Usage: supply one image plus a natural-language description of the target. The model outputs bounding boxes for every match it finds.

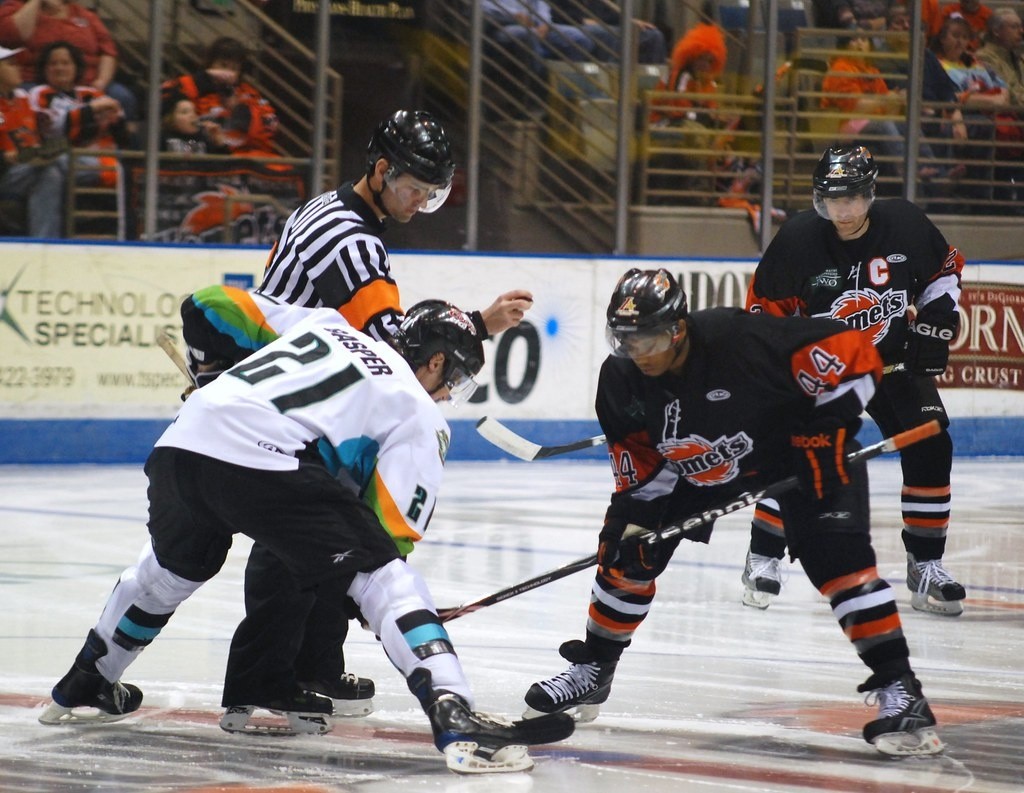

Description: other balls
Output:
[514,297,534,312]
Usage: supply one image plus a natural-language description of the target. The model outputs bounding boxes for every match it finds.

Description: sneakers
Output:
[521,640,624,722]
[407,668,534,774]
[219,687,333,736]
[302,671,376,718]
[856,667,946,757]
[905,551,966,616]
[38,627,144,724]
[740,547,786,609]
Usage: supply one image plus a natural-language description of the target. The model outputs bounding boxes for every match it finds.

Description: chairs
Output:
[405,1,855,202]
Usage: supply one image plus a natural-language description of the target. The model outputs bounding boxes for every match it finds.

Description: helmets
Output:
[397,298,485,386]
[811,145,879,203]
[365,109,456,190]
[605,266,688,339]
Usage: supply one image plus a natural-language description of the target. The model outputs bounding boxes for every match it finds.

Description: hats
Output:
[1,45,25,60]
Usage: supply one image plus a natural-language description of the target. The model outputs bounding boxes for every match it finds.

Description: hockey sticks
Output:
[376,420,942,641]
[156,334,575,746]
[475,362,905,463]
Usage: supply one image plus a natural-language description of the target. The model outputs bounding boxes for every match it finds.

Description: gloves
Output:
[180,351,237,401]
[785,414,852,503]
[595,492,684,581]
[903,309,959,376]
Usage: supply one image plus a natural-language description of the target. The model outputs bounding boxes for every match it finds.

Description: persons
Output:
[221,107,533,737]
[522,268,947,758]
[741,139,965,616]
[463,0,1024,208]
[36,286,546,775]
[0,0,304,237]
